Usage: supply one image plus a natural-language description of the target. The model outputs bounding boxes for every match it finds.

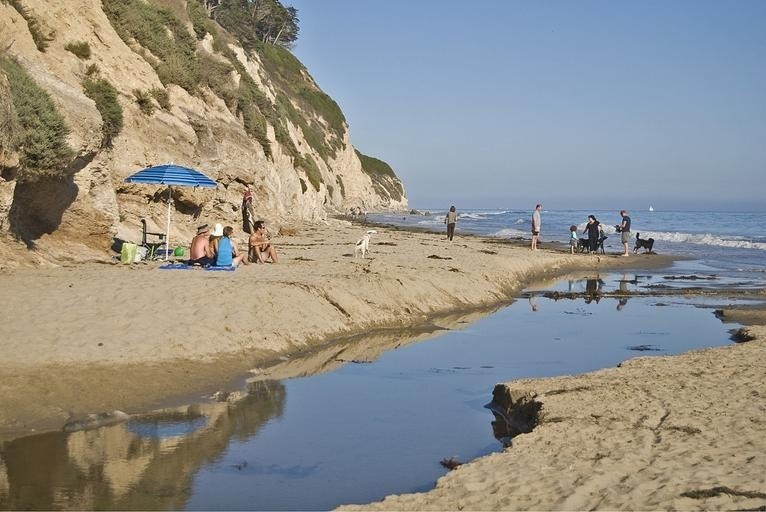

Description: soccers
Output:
[174,248,183,256]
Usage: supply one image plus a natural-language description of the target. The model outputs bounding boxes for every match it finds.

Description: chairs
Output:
[141,219,167,261]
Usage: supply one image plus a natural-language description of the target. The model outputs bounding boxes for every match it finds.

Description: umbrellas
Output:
[123,161,218,260]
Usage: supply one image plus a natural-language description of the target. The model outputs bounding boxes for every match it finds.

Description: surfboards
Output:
[245,190,255,232]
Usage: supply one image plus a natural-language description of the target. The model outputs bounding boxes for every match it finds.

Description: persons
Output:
[568,224,580,255]
[247,220,280,265]
[209,222,223,266]
[530,203,543,251]
[188,222,215,266]
[443,205,457,241]
[584,280,597,304]
[215,226,249,269]
[614,272,630,311]
[528,296,538,312]
[582,214,603,255]
[618,209,631,257]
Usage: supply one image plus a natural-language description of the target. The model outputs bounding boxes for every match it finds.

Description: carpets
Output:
[157,261,239,272]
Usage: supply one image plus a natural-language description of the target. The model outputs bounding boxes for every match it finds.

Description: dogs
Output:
[633,232,654,256]
[354,234,370,258]
[579,238,590,253]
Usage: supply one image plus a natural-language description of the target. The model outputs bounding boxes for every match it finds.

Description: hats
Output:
[196,223,223,237]
